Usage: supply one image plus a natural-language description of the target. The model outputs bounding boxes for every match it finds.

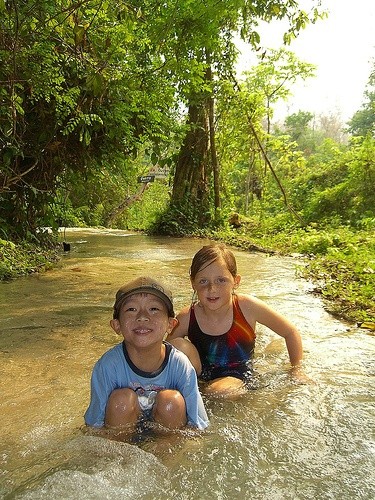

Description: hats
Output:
[113,276,176,317]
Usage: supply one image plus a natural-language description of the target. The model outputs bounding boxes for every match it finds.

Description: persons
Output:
[83,277,210,433]
[165,241,303,399]
